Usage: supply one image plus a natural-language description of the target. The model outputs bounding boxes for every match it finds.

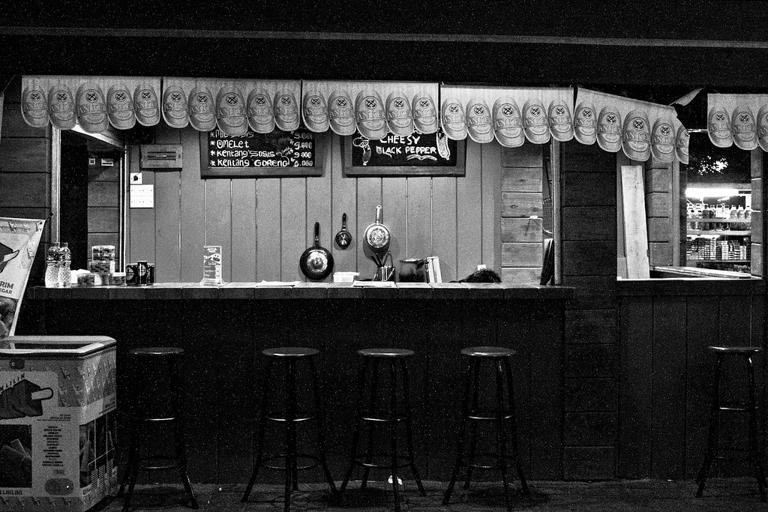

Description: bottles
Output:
[45,242,71,289]
[687,203,752,260]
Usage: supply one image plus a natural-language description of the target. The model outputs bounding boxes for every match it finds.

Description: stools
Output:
[240,347,337,512]
[440,344,530,512]
[337,348,427,512]
[120,346,199,512]
[695,344,768,503]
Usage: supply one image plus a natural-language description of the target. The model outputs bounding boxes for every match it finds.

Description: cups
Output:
[78,273,95,286]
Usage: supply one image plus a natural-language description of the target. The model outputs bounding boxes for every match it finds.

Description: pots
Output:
[300,205,390,281]
[399,258,426,281]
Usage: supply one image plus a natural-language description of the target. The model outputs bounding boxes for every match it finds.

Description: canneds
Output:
[126,260,155,287]
